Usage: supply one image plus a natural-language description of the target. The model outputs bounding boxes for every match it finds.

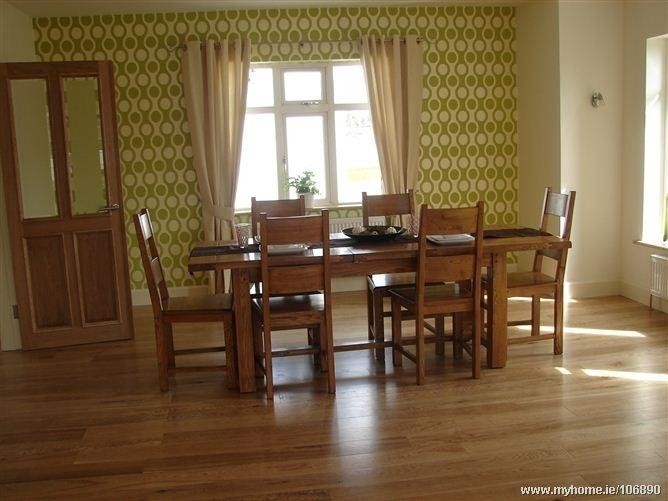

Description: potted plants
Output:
[286,170,320,210]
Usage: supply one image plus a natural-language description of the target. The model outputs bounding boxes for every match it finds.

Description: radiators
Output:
[226,216,386,296]
[648,255,668,311]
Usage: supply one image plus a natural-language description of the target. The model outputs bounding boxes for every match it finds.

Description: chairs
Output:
[386,201,485,387]
[251,196,319,348]
[361,190,447,362]
[132,208,239,393]
[456,184,577,369]
[248,210,338,399]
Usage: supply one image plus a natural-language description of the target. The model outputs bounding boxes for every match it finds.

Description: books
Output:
[425,234,474,244]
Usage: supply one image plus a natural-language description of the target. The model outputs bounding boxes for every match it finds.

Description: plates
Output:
[341,225,406,240]
[425,234,476,246]
[258,244,308,254]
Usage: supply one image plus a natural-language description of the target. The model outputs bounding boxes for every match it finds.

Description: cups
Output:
[233,222,250,249]
